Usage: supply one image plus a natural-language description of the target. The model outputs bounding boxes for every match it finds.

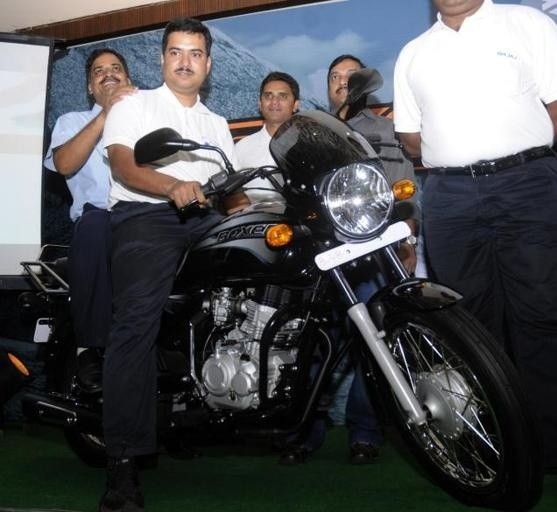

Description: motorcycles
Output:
[0,66,531,504]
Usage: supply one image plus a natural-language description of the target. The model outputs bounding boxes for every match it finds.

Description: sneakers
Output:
[279,441,319,466]
[98,456,145,512]
[347,441,385,465]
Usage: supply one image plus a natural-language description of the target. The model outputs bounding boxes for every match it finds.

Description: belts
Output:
[426,146,554,176]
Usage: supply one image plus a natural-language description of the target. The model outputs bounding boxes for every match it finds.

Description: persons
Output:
[385,1,556,484]
[95,11,261,512]
[233,67,305,202]
[278,51,423,474]
[41,46,146,468]
